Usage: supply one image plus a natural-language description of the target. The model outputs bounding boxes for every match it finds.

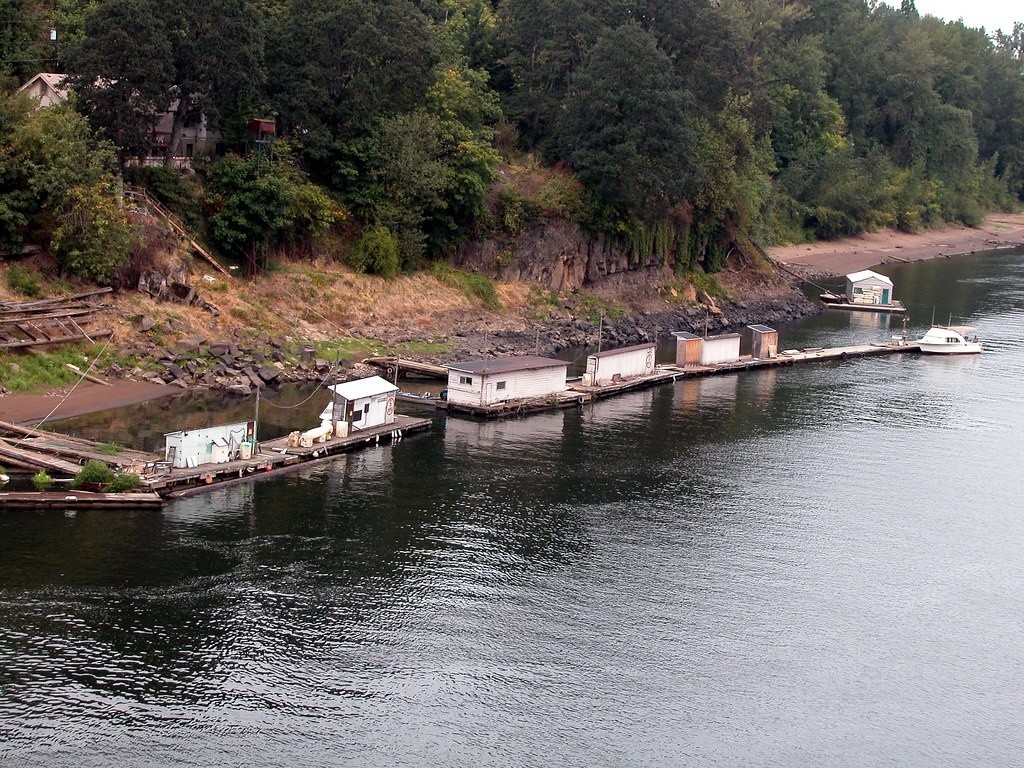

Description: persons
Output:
[964,335,977,343]
[125,188,142,203]
[172,227,185,242]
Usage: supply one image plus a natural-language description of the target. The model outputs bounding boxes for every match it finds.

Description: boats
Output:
[916,325,984,356]
